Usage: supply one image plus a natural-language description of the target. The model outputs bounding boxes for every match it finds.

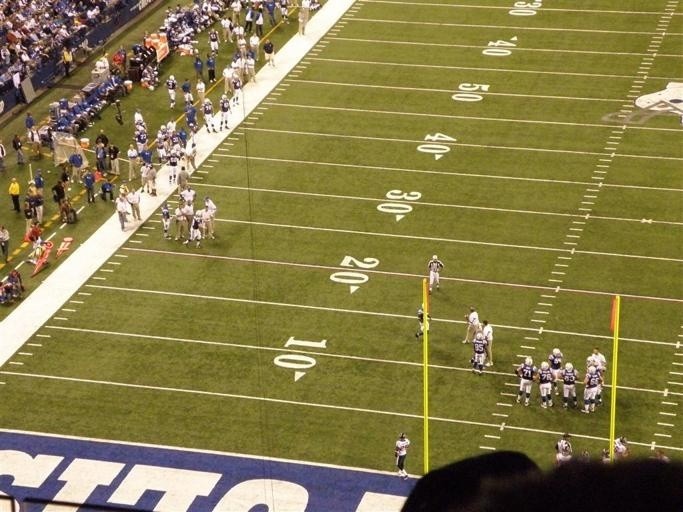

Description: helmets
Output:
[476,333,597,374]
[399,432,405,439]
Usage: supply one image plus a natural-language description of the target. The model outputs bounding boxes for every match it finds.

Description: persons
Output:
[395,433,410,481]
[555,433,630,481]
[509,347,607,415]
[416,303,431,339]
[0,1,321,303]
[428,255,444,295]
[656,450,670,463]
[462,307,494,376]
